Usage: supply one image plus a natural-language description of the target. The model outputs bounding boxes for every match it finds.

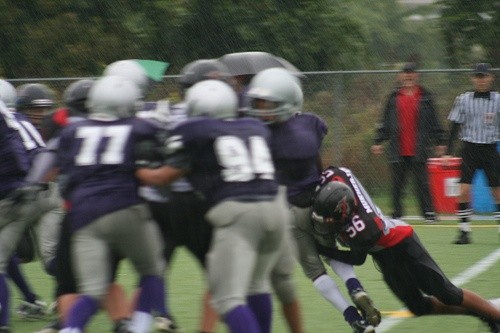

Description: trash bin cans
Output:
[427,157,463,214]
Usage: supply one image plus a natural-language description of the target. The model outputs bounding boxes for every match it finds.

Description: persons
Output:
[371,62,445,223]
[293,167,500,333]
[0,59,380,333]
[443,61,500,244]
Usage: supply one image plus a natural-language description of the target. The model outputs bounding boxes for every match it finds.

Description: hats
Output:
[472,62,493,75]
[401,64,416,73]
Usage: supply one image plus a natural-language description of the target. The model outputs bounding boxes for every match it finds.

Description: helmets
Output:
[85,75,142,122]
[176,59,234,85]
[311,180,355,235]
[103,60,150,98]
[183,79,239,121]
[0,79,15,104]
[14,84,57,113]
[243,67,304,125]
[63,79,92,110]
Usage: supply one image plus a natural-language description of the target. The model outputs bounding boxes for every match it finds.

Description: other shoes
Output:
[452,231,469,244]
[424,211,436,220]
[351,288,381,327]
[351,319,376,333]
[155,316,174,332]
[47,300,58,315]
[17,301,45,320]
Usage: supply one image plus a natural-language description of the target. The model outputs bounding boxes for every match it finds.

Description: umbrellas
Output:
[219,52,307,77]
